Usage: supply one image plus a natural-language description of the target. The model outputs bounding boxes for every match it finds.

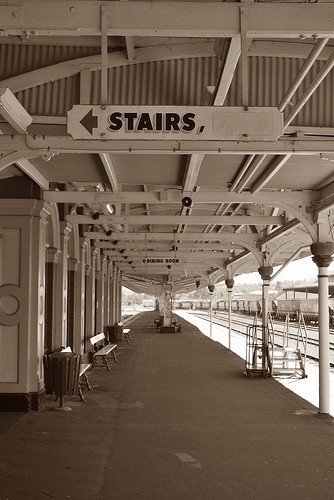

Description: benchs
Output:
[153,316,182,333]
[60,345,93,402]
[89,332,119,371]
[118,321,133,344]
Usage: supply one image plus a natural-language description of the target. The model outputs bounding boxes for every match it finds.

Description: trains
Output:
[142,299,334,324]
